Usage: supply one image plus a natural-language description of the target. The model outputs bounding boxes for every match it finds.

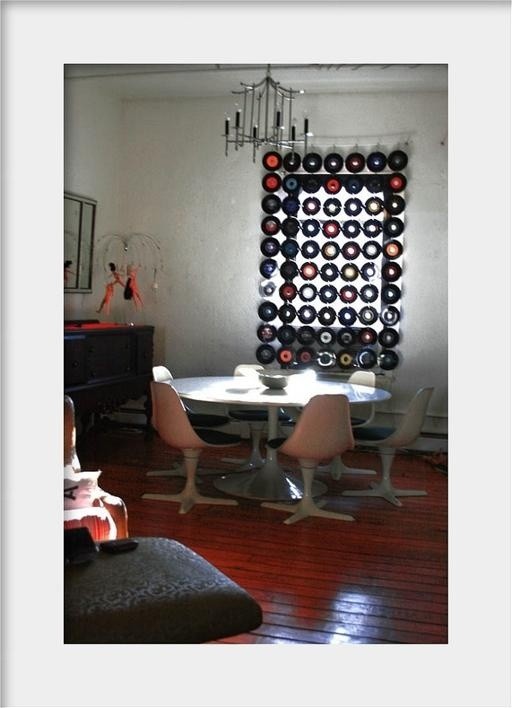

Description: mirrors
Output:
[65,193,99,294]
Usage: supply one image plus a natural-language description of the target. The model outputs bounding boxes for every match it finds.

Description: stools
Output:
[65,536,264,642]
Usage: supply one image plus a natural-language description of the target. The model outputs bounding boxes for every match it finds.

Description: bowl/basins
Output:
[254,368,306,390]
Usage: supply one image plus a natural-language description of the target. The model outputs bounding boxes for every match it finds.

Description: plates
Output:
[256,148,407,372]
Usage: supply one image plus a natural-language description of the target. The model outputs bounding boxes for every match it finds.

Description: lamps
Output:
[220,64,314,165]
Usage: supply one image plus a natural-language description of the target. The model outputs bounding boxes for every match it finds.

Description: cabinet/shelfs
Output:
[64,318,155,445]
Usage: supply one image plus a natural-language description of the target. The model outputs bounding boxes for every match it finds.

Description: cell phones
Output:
[100,539,138,553]
